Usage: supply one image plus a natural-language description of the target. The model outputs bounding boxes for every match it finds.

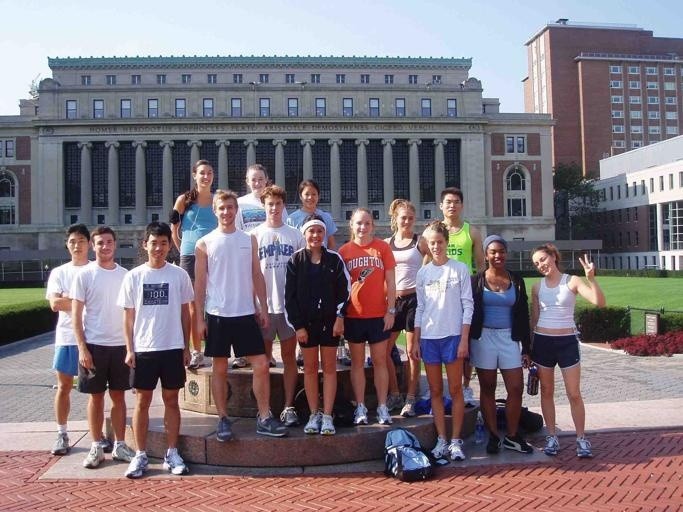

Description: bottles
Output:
[527,367,540,395]
[337,336,348,359]
[473,411,485,443]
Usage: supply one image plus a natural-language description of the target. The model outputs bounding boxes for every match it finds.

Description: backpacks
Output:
[384,427,436,482]
[414,397,452,415]
[494,399,543,432]
[292,384,359,426]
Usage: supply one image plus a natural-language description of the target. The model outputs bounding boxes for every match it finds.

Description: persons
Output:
[170,159,219,368]
[469,235,535,454]
[288,179,352,365]
[423,187,487,408]
[381,198,433,417]
[232,163,289,368]
[117,222,196,479]
[411,220,474,462]
[45,224,111,454]
[283,214,351,436]
[249,186,305,427]
[529,244,606,458]
[337,207,397,426]
[194,190,289,443]
[69,225,136,468]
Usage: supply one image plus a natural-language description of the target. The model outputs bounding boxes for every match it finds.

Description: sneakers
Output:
[386,394,404,411]
[448,439,466,461]
[232,357,246,368]
[544,436,560,455]
[304,412,324,433]
[337,349,351,365]
[112,444,136,462]
[320,415,336,435]
[270,357,276,367]
[83,446,105,468]
[280,406,300,426]
[376,406,393,425]
[430,437,451,458]
[503,435,533,453]
[576,437,593,458]
[188,351,204,369]
[126,453,149,478]
[163,451,190,474]
[487,434,502,453]
[51,433,69,454]
[463,387,475,407]
[256,415,289,437]
[296,350,303,366]
[353,404,368,424]
[400,398,416,416]
[217,416,232,442]
[100,436,110,451]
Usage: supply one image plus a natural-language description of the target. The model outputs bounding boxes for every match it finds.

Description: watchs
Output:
[336,312,344,317]
[386,307,396,313]
